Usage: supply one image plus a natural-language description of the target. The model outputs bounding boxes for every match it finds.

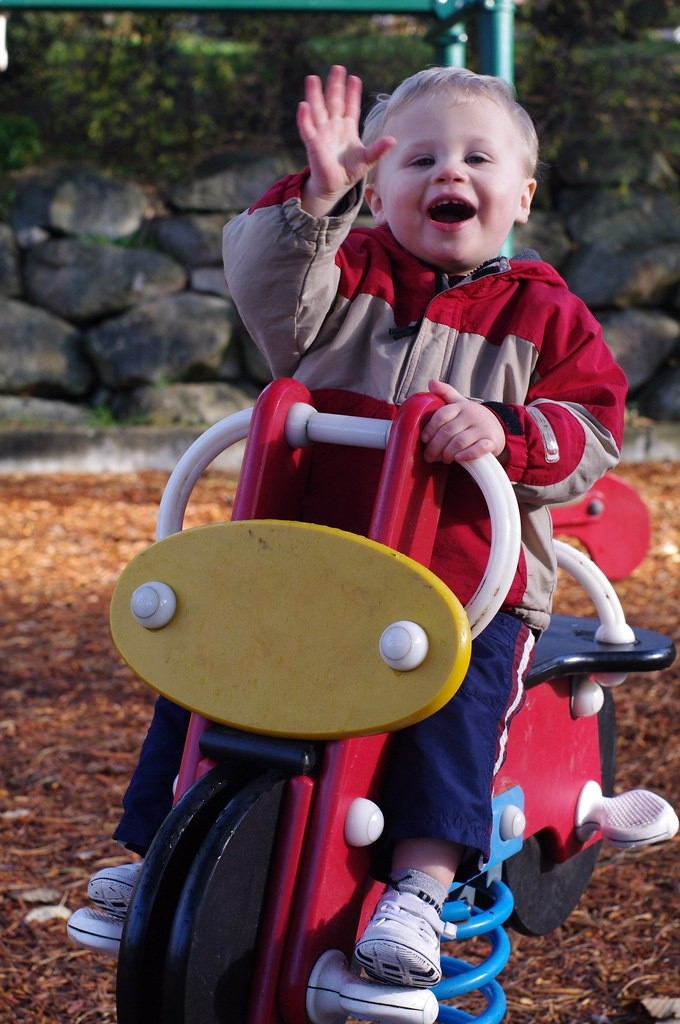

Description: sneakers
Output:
[85,862,144,919]
[352,891,458,990]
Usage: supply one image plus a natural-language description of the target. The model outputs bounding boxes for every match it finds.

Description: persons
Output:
[88,66,628,986]
